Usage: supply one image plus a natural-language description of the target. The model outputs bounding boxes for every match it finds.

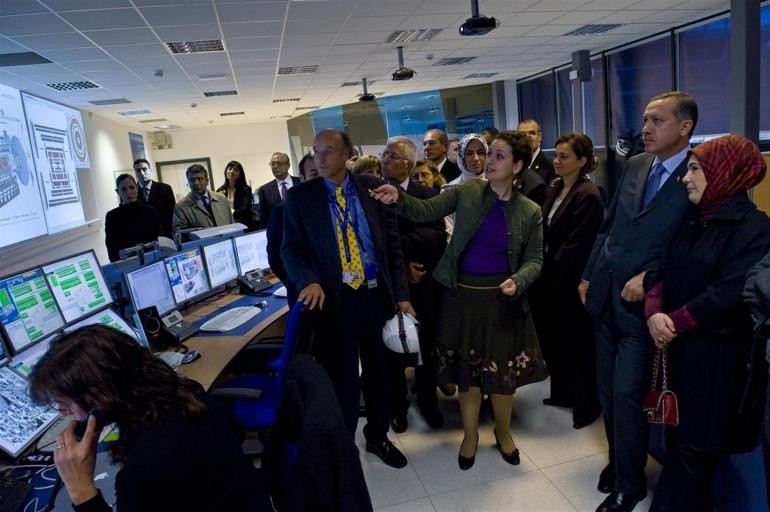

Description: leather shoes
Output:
[494,427,520,465]
[391,414,407,433]
[597,464,616,493]
[366,436,407,468]
[420,405,444,429]
[458,433,479,470]
[595,484,647,511]
[439,382,455,396]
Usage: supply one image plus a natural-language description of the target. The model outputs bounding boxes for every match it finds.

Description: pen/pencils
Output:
[367,185,402,206]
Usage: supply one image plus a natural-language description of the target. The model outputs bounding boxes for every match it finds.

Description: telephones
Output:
[159,308,199,345]
[237,267,273,292]
[73,405,108,443]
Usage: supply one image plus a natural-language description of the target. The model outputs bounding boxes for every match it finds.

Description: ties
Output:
[282,181,287,199]
[143,187,149,201]
[334,186,366,291]
[642,162,666,210]
[201,194,212,214]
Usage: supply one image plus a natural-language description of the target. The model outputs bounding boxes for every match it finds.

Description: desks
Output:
[35,273,291,511]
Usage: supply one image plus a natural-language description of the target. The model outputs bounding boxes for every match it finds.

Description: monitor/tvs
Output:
[0,248,146,458]
[200,236,241,290]
[100,226,205,308]
[232,228,273,277]
[164,244,213,310]
[120,258,180,318]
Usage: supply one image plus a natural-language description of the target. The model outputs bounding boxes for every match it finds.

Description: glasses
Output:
[269,161,287,167]
[378,152,410,163]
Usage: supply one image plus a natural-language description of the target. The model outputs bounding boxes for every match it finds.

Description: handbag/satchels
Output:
[641,389,680,426]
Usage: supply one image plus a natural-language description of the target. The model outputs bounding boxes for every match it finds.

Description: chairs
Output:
[212,289,313,426]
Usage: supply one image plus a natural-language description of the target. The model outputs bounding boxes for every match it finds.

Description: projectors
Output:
[392,68,414,80]
[459,18,495,36]
[359,93,374,101]
